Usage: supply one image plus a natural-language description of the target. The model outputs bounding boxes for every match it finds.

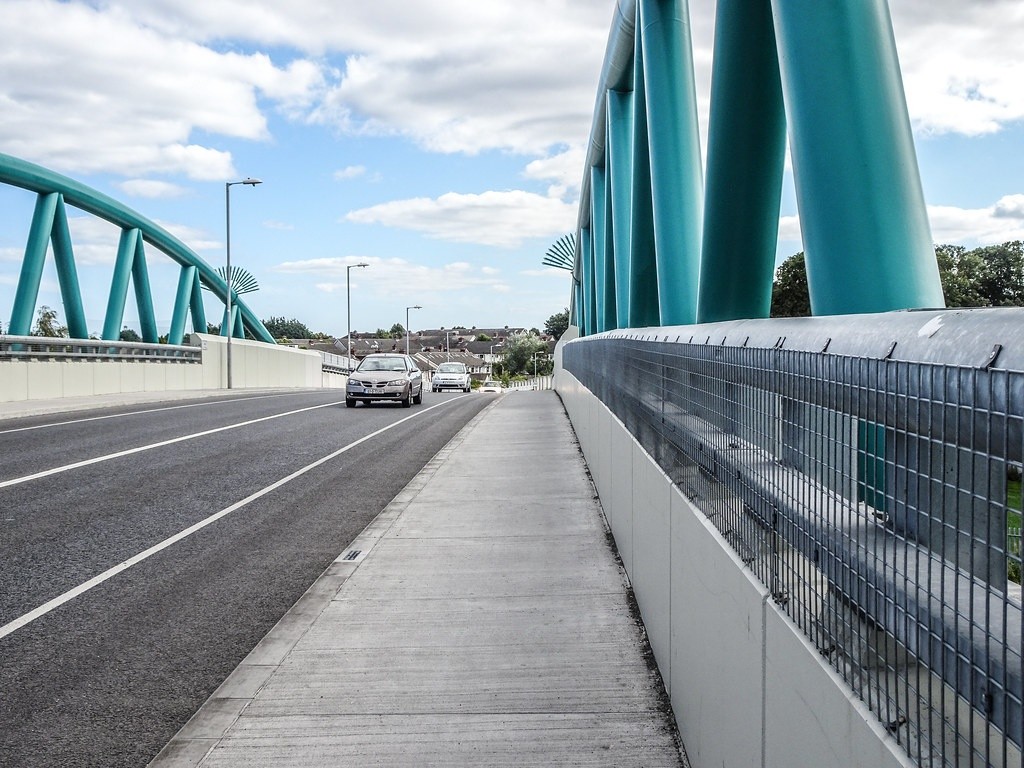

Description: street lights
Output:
[406,305,423,355]
[347,261,370,376]
[535,351,545,379]
[225,177,264,390]
[446,330,459,362]
[490,343,502,382]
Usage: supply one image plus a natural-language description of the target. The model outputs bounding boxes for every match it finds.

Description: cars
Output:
[346,352,423,408]
[431,361,471,393]
